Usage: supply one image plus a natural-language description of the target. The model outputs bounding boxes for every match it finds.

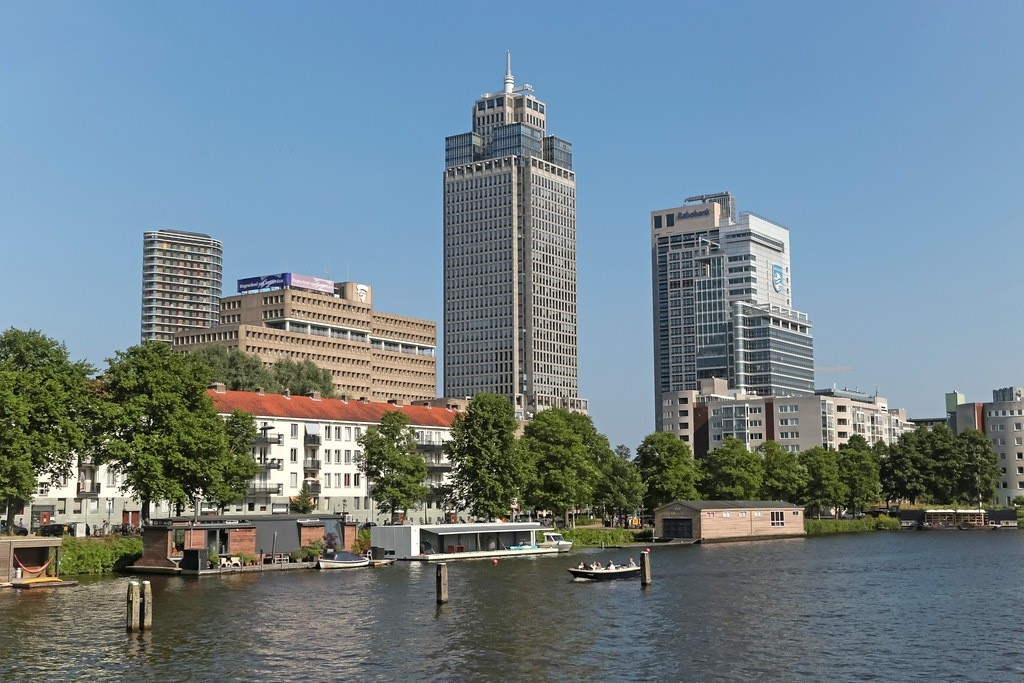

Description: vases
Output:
[257,562,260,565]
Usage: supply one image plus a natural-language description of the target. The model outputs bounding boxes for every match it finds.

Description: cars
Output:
[35,524,74,537]
[111,525,131,536]
[0,519,28,536]
[556,520,572,529]
[839,506,899,521]
[363,521,377,530]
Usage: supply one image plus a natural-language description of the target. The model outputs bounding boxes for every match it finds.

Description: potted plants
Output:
[302,539,324,562]
[292,550,307,562]
[323,532,338,553]
[243,555,257,566]
[352,538,369,557]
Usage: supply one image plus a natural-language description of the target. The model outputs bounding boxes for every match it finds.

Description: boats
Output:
[567,565,642,581]
[899,519,1003,531]
[318,554,371,569]
[534,531,574,552]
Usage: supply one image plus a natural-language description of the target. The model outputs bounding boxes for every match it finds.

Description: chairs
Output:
[219,558,230,567]
[230,557,240,567]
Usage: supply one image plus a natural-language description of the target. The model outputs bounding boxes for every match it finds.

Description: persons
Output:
[119,523,140,535]
[602,513,655,528]
[18,518,24,528]
[577,555,636,570]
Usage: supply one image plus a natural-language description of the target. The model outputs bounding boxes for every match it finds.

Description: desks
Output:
[447,545,465,553]
